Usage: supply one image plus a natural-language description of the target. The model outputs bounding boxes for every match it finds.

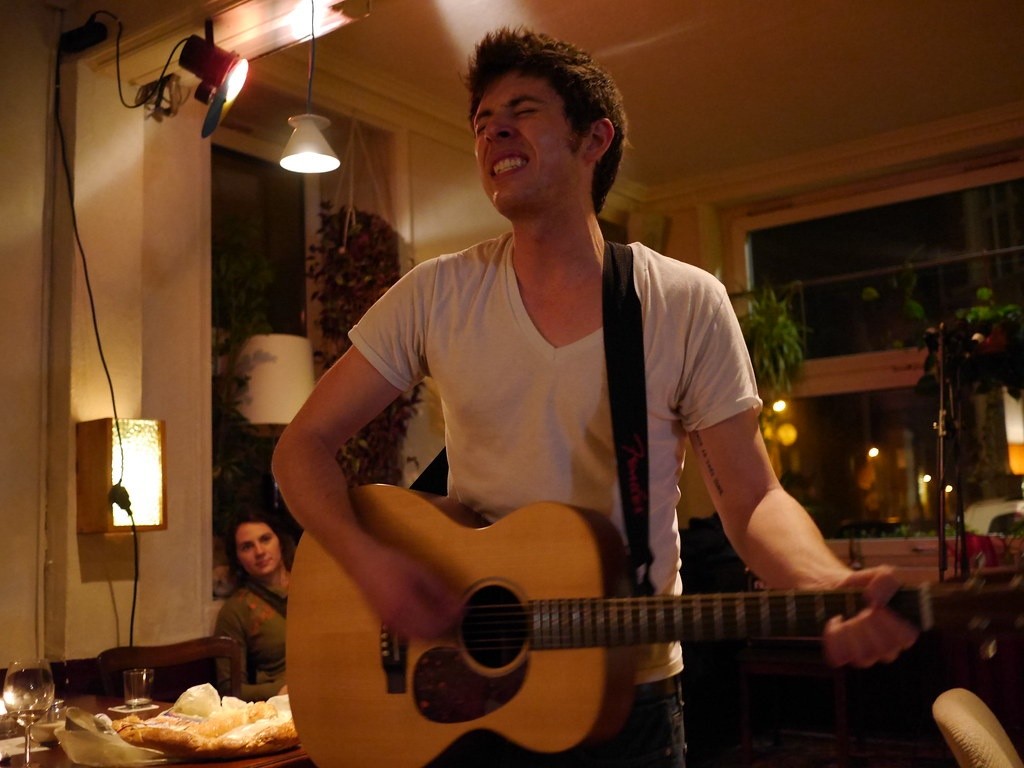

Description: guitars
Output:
[283,480,1021,768]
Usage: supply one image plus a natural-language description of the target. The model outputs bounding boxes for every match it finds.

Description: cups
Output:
[30,698,65,743]
[123,667,155,708]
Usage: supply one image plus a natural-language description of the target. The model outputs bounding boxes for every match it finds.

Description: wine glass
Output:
[4,658,56,768]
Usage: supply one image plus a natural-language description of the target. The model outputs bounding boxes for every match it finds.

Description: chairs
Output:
[96,636,247,703]
[930,680,1024,768]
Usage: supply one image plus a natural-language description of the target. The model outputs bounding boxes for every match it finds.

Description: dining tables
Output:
[0,695,315,768]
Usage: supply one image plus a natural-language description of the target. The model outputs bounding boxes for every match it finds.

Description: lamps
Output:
[76,415,168,535]
[280,0,341,175]
[177,14,251,107]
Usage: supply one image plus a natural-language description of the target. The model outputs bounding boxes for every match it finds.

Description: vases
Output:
[231,332,316,424]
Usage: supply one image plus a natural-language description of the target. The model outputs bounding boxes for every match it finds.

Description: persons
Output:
[271,23,921,768]
[211,510,291,704]
